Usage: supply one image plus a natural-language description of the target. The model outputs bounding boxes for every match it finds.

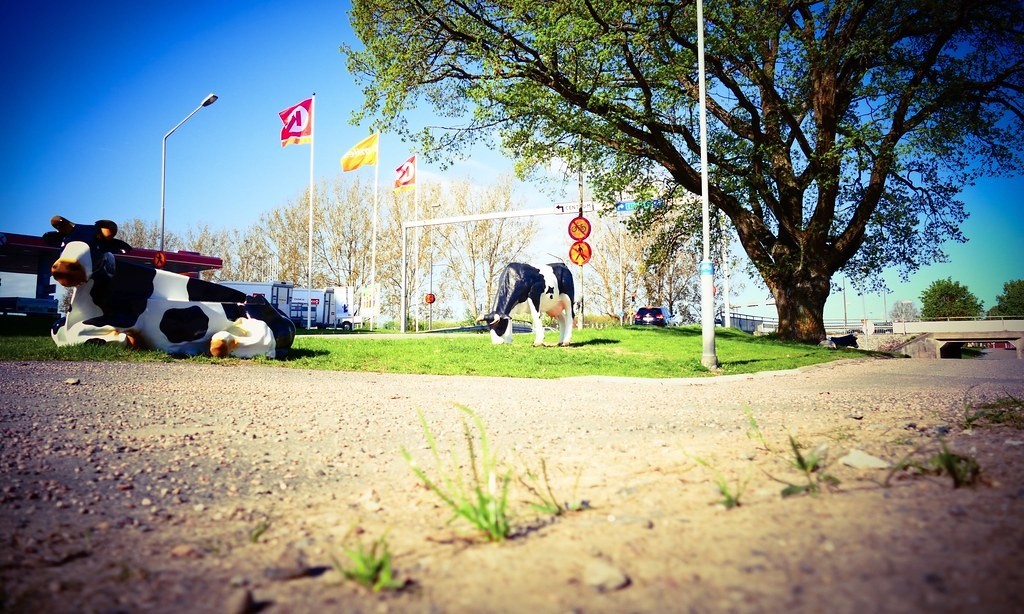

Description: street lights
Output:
[429,204,441,330]
[159,94,219,250]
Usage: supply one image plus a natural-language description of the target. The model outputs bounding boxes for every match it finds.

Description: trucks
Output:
[217,280,293,319]
[289,288,336,330]
[334,287,364,330]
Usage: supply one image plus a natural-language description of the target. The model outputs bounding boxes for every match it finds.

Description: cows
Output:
[826,334,859,348]
[475,262,575,346]
[46,216,295,359]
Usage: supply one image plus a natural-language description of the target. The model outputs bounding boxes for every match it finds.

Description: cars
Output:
[634,306,676,328]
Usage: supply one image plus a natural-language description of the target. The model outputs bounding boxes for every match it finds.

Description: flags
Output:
[340,133,377,172]
[278,99,312,147]
[394,156,415,188]
